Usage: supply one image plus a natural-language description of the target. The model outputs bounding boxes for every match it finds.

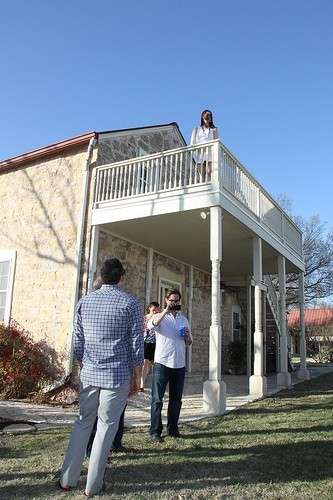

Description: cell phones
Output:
[170,305,181,310]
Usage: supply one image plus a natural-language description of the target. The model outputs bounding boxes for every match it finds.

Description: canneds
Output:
[180,326,185,337]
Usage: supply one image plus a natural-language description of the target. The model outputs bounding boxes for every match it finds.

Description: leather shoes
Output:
[112,447,137,454]
[153,435,164,444]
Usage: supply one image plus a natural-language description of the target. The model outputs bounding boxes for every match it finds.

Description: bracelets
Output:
[186,339,191,345]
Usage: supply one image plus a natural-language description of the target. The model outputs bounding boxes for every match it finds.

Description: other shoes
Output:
[139,388,145,392]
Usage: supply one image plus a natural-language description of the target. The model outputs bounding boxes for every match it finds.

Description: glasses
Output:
[168,298,180,303]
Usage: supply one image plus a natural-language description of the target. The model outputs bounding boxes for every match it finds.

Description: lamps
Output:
[200,210,211,220]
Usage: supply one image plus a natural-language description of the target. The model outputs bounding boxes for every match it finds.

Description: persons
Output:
[139,302,159,393]
[86,276,137,465]
[147,288,193,444]
[57,258,145,497]
[190,110,219,182]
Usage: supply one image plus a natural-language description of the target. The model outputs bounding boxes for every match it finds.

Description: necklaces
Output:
[203,127,211,139]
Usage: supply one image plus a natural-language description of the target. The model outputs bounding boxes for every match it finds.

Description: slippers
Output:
[56,475,71,491]
[84,480,106,498]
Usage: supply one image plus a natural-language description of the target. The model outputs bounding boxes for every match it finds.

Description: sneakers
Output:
[169,434,187,440]
